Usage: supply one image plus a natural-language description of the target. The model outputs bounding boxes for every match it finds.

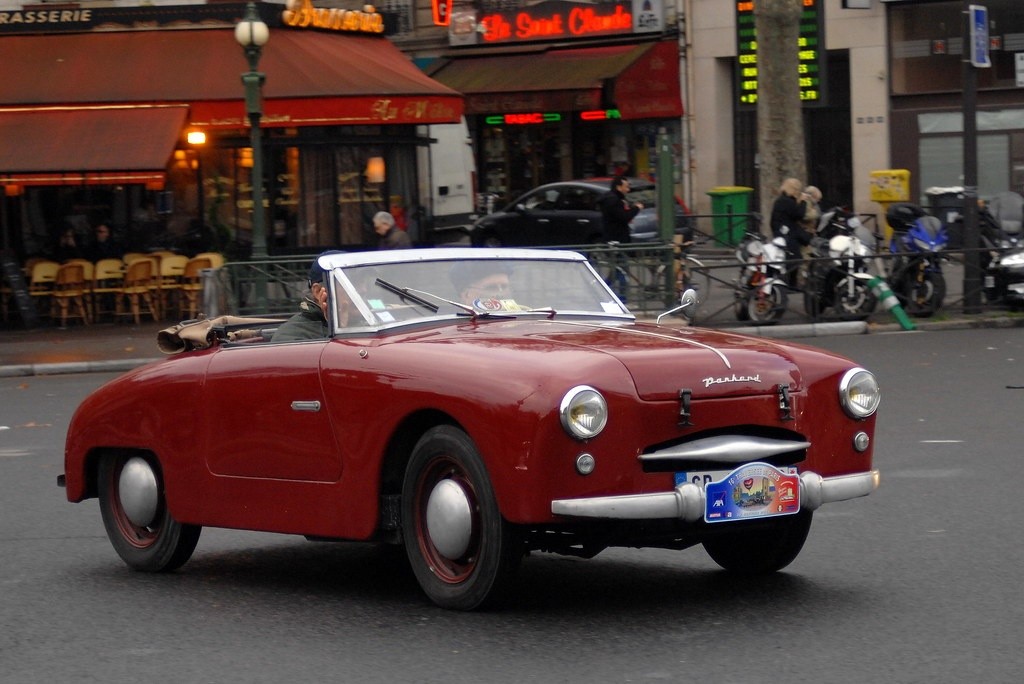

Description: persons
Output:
[770,178,809,293]
[600,176,643,309]
[270,250,383,343]
[451,261,512,304]
[798,186,823,287]
[595,155,607,178]
[50,224,122,262]
[373,211,411,250]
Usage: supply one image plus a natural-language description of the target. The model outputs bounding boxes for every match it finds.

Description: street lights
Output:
[235,0,277,315]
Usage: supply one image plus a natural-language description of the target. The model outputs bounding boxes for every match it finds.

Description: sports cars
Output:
[57,247,884,616]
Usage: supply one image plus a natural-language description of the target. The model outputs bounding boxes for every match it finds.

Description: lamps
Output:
[363,156,386,184]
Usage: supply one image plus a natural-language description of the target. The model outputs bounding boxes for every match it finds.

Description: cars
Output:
[469,175,696,261]
[983,230,1024,311]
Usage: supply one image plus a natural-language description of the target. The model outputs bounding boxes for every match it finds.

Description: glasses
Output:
[467,283,512,295]
[373,222,383,231]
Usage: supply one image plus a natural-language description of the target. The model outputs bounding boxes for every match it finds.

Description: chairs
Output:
[4,249,229,332]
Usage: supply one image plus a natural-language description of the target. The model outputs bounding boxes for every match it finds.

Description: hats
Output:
[308,250,381,288]
[448,256,513,291]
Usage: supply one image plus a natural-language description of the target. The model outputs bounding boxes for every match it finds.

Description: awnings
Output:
[0,106,190,184]
[0,30,462,131]
[425,40,684,119]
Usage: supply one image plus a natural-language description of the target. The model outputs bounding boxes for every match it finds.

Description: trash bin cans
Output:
[924,186,967,249]
[705,186,754,247]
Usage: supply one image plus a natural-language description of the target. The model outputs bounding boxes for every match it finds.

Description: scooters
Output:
[732,223,798,325]
[883,200,959,318]
[796,206,889,321]
